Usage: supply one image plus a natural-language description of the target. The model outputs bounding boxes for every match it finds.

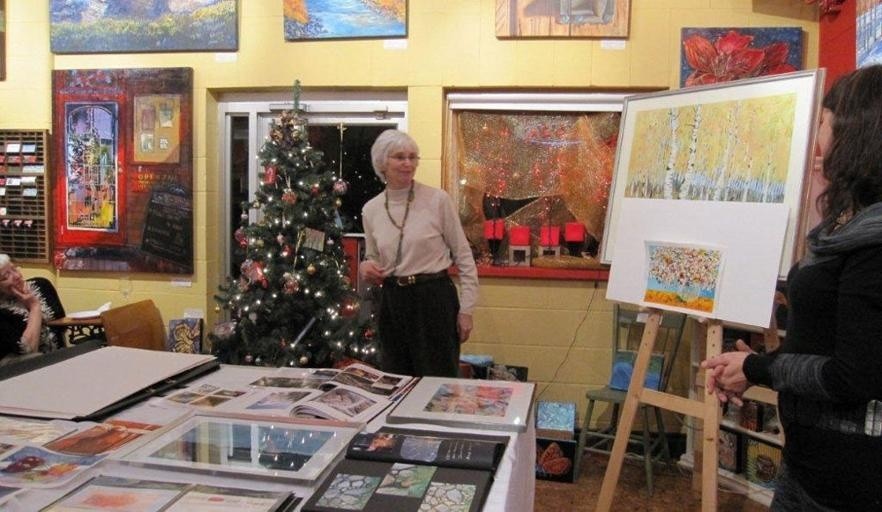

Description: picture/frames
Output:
[598,65,829,283]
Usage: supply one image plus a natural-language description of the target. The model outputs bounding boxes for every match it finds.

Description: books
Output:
[608,348,665,392]
[537,436,578,486]
[718,327,782,492]
[533,400,575,437]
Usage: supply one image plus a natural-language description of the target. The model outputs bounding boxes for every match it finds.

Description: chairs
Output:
[572,301,691,495]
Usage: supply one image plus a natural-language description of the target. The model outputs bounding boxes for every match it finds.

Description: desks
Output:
[2,344,540,512]
[46,312,104,345]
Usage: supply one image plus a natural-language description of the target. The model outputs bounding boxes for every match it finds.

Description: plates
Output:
[68,310,101,320]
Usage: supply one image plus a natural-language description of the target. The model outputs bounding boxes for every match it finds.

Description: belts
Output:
[382,269,449,287]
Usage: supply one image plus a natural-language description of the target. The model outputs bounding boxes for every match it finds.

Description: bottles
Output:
[743,399,765,433]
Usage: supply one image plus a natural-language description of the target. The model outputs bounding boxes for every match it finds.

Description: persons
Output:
[356,128,481,376]
[0,254,69,360]
[700,66,881,511]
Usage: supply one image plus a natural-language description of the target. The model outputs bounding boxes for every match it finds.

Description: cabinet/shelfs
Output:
[681,315,796,507]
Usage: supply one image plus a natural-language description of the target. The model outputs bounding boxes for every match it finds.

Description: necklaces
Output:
[385,180,415,264]
